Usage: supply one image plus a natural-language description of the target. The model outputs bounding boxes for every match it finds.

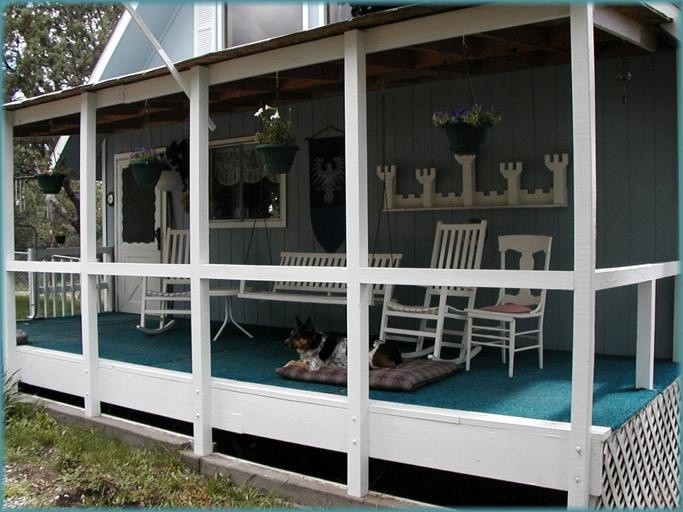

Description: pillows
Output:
[275,359,464,393]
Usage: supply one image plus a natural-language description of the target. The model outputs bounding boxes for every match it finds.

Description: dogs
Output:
[281,312,402,372]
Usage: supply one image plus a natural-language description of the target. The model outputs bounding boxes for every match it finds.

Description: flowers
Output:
[26,125,68,175]
[125,143,163,163]
[430,103,502,129]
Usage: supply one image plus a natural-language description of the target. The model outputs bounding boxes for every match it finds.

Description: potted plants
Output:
[253,102,300,175]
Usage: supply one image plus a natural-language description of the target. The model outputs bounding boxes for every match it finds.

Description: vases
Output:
[128,163,163,190]
[34,174,67,194]
[441,123,489,156]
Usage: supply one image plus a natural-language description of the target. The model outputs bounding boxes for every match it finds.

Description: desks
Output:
[209,286,256,343]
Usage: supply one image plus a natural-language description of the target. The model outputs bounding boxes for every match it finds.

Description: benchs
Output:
[237,251,403,309]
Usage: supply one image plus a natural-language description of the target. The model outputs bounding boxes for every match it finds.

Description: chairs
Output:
[135,226,191,336]
[462,233,553,380]
[376,218,489,367]
